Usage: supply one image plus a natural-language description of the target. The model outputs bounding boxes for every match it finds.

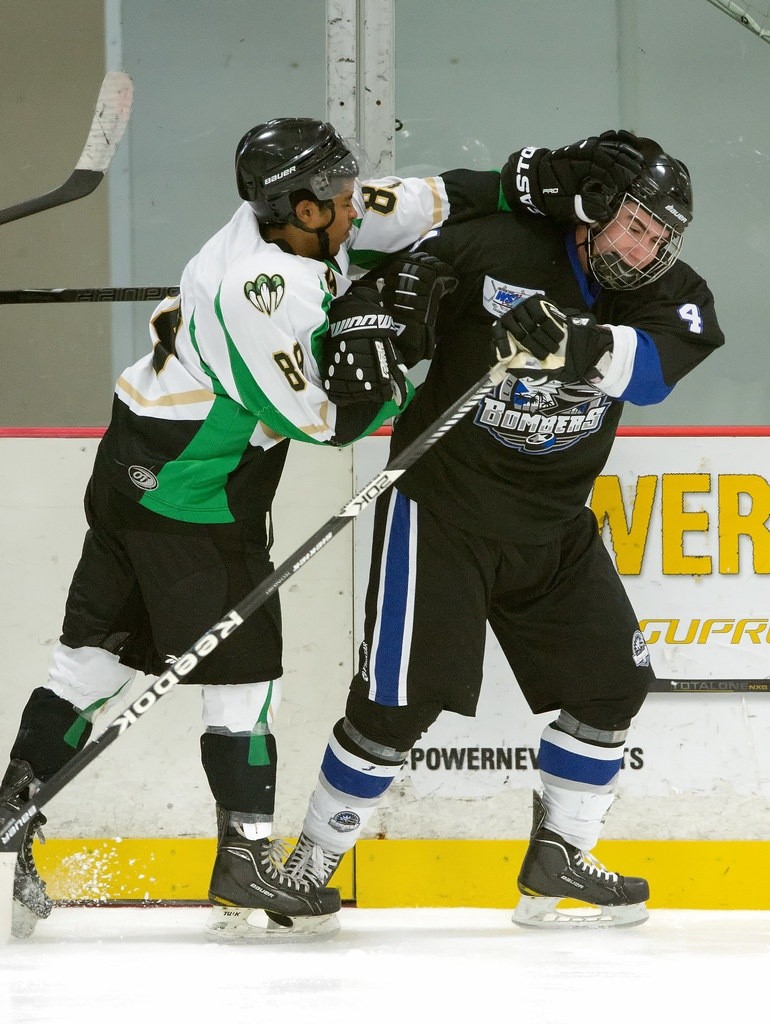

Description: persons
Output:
[0,118,648,939]
[256,137,727,930]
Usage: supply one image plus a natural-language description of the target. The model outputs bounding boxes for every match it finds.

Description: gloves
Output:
[502,137,644,223]
[489,294,612,384]
[324,286,409,410]
[373,252,460,328]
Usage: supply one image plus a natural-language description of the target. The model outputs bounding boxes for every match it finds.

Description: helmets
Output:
[587,136,693,291]
[235,117,372,233]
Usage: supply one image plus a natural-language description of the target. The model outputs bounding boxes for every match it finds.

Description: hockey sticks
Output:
[0,287,179,305]
[0,309,563,944]
[1,69,135,226]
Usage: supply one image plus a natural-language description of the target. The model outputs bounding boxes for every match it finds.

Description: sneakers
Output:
[0,758,53,940]
[511,789,649,928]
[208,802,342,945]
[264,831,346,927]
